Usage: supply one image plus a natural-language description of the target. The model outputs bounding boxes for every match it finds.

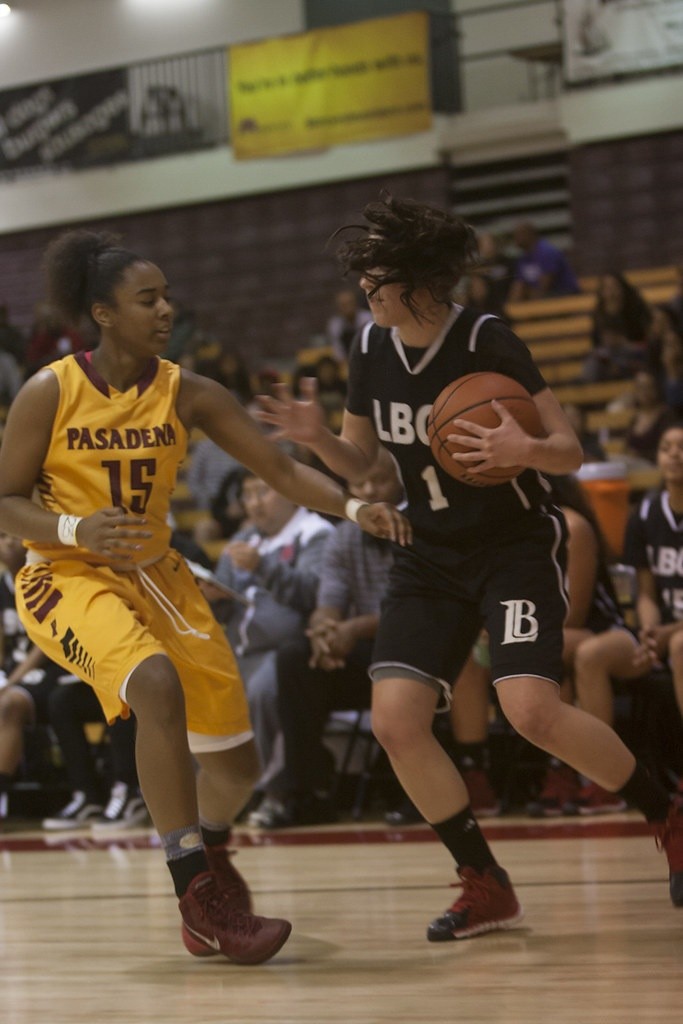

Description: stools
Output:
[333,692,383,816]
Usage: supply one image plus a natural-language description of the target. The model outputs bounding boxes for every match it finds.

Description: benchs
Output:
[0,264,682,741]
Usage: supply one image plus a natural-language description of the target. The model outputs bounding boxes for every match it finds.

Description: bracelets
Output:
[57,514,83,547]
[345,498,369,524]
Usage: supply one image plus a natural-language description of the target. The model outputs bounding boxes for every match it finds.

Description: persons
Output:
[256,193,683,941]
[0,231,415,964]
[0,219,683,829]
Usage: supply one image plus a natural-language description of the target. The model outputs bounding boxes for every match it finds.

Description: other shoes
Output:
[525,790,579,817]
[562,787,628,816]
[384,805,420,825]
[249,799,288,830]
[469,796,503,818]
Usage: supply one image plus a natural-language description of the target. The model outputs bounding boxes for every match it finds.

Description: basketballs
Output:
[427,371,543,489]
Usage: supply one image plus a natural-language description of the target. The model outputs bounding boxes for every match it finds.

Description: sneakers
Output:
[90,780,150,832]
[178,869,293,966]
[650,794,683,908]
[41,790,105,831]
[203,842,253,914]
[426,863,525,942]
